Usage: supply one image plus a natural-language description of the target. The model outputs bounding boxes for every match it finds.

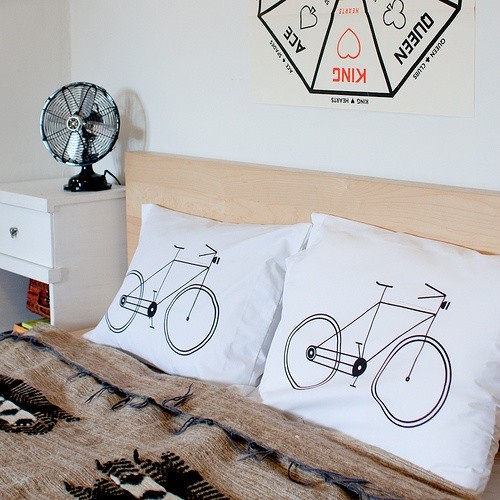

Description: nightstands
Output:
[0,176,130,333]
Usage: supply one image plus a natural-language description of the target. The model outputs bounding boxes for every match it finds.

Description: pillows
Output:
[78,199,317,393]
[220,210,500,498]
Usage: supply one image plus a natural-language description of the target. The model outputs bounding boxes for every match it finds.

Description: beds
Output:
[0,324,500,500]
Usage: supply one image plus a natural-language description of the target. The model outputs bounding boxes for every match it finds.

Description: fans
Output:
[39,81,121,193]
